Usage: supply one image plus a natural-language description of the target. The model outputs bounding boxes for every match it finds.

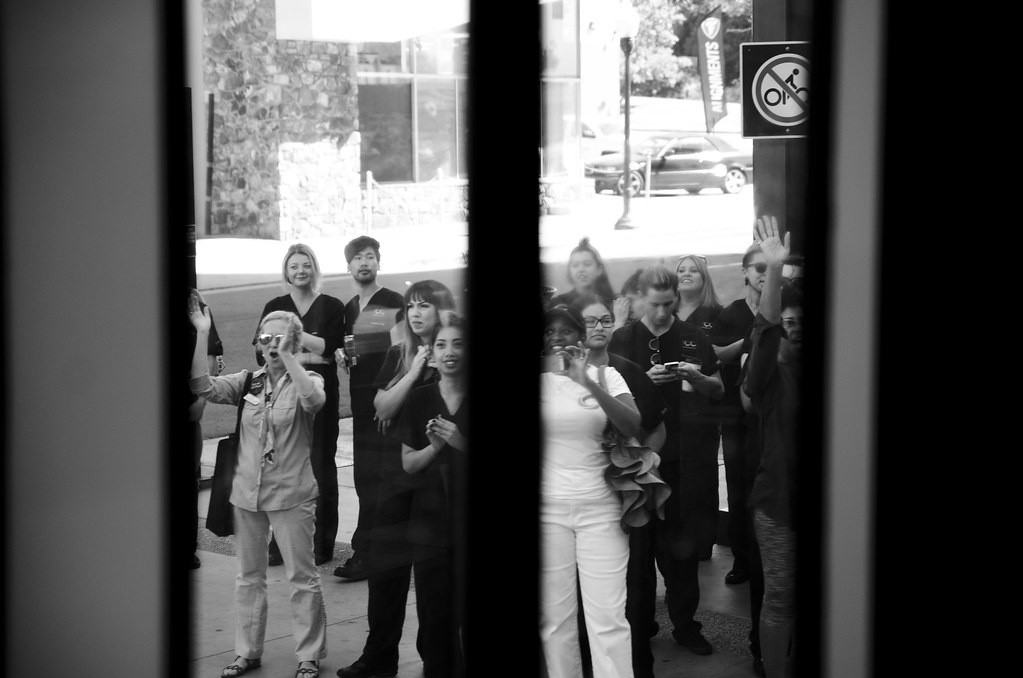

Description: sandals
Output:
[221,655,260,677]
[295,660,320,678]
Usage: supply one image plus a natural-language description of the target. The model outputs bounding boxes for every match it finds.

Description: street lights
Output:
[613,7,640,231]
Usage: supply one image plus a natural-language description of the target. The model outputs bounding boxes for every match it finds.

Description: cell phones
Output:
[542,355,565,373]
[664,362,678,370]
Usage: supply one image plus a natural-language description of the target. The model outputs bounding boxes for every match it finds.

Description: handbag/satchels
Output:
[205,373,253,537]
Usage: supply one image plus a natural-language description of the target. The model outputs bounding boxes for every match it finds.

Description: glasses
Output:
[679,255,707,265]
[649,337,661,365]
[748,263,767,273]
[583,315,615,328]
[258,334,282,345]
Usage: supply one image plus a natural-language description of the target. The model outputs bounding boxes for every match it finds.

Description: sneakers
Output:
[337,656,398,678]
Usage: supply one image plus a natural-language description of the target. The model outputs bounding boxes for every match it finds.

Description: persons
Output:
[607,267,724,657]
[550,238,618,311]
[332,236,406,582]
[673,254,725,339]
[570,293,666,678]
[336,280,456,678]
[611,269,648,330]
[252,243,345,566]
[717,214,803,678]
[542,303,640,678]
[193,297,326,678]
[193,280,225,577]
[401,309,463,678]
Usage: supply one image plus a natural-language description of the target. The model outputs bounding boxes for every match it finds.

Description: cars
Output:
[584,133,753,197]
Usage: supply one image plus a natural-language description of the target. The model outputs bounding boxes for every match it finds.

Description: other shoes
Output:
[676,633,714,655]
[333,554,370,578]
[726,569,748,584]
[269,549,284,567]
[190,554,201,568]
[697,544,713,560]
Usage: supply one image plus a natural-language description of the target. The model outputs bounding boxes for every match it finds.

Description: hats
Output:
[544,303,585,333]
